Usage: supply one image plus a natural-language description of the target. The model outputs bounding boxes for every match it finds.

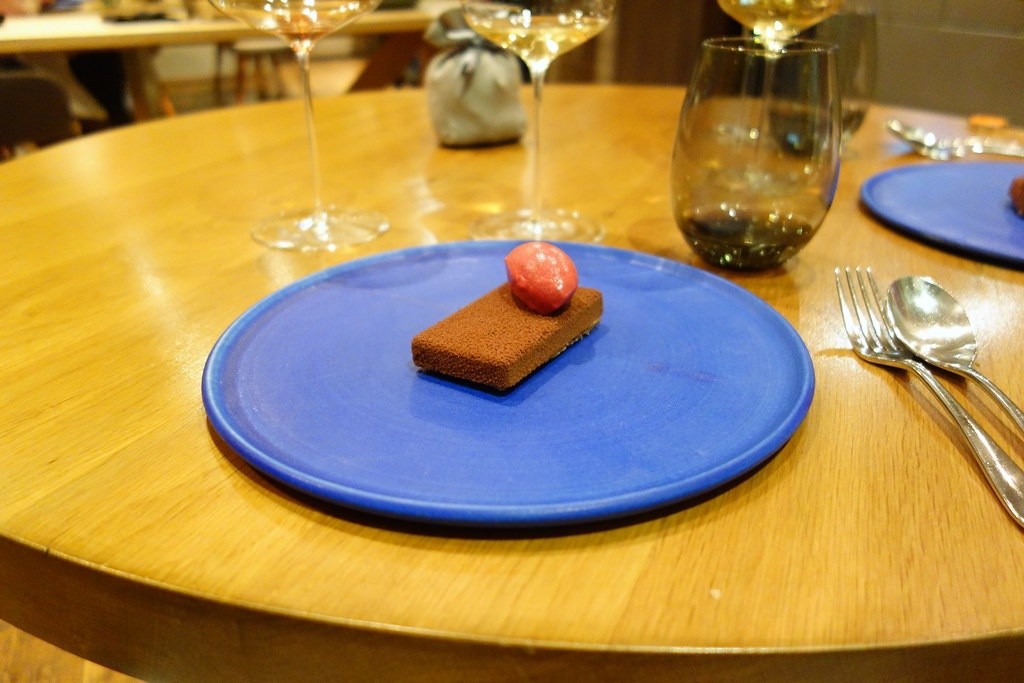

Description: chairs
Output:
[213,15,291,105]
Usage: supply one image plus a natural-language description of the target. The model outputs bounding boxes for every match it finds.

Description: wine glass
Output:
[716,1,842,153]
[454,1,615,244]
[207,1,390,251]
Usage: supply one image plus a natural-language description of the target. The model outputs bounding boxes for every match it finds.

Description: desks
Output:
[0,10,433,54]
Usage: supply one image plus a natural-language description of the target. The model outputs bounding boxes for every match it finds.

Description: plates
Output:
[200,238,816,530]
[861,159,1024,268]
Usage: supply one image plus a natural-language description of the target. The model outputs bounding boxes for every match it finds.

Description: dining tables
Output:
[0,83,1024,654]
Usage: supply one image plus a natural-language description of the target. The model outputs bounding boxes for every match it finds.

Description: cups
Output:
[670,37,842,272]
[767,0,880,162]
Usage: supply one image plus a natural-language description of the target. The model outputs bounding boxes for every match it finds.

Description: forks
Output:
[835,266,1024,528]
[909,142,1024,161]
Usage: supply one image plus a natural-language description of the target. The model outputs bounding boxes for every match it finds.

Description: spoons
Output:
[886,122,1024,150]
[885,275,1024,435]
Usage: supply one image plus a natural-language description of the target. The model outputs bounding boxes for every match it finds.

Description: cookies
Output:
[411,279,603,389]
